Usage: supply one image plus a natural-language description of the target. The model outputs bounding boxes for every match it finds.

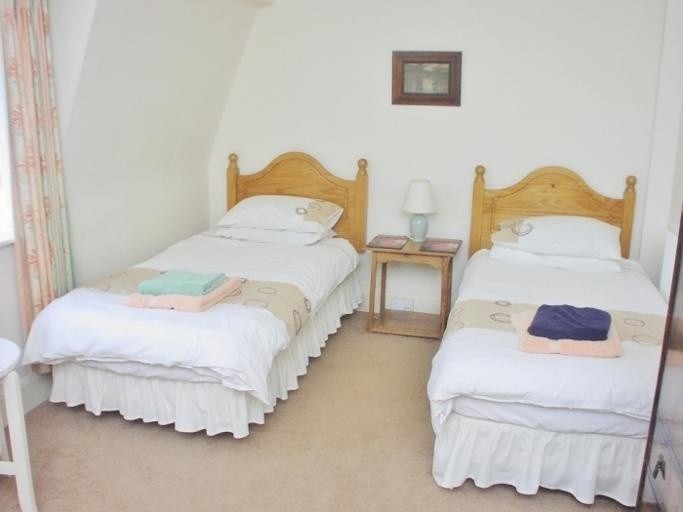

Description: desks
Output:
[0,336,38,512]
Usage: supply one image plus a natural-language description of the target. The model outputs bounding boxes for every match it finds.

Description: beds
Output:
[425,161,669,509]
[35,150,370,446]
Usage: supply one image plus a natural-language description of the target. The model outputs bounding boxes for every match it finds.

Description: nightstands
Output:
[364,231,449,339]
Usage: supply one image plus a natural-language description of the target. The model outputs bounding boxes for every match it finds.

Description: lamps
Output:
[402,178,439,242]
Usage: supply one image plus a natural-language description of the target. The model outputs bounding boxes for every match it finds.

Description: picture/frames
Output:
[390,49,463,106]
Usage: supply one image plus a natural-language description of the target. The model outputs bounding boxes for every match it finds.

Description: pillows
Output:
[489,212,625,262]
[215,226,341,246]
[214,193,345,235]
[484,244,624,275]
